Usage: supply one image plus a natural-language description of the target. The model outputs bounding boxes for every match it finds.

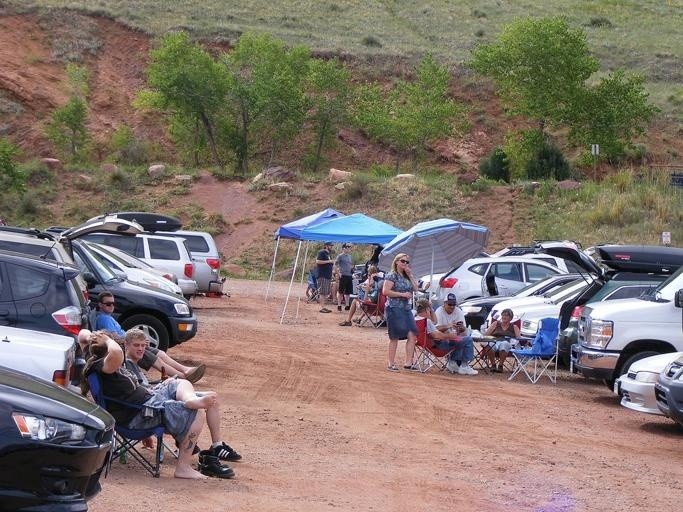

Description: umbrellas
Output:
[378,217,492,303]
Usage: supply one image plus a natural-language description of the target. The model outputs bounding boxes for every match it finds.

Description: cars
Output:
[0,326,76,391]
[612,351,682,417]
[0,369,116,512]
[655,352,682,431]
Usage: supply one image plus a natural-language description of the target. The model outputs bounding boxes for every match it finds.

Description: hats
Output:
[444,293,456,302]
[324,242,334,246]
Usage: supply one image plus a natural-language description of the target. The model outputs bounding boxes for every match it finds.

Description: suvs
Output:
[568,264,682,390]
[0,252,91,341]
[557,243,682,370]
[0,210,226,358]
[418,239,604,334]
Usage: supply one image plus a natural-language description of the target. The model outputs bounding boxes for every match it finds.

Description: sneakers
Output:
[387,365,398,372]
[403,363,417,370]
[319,306,349,312]
[176,440,241,477]
[353,319,360,324]
[339,321,352,326]
[446,362,503,374]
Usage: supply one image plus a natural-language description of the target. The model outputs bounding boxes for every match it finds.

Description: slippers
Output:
[184,364,206,384]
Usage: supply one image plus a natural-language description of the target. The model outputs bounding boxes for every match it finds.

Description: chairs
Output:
[307,271,560,384]
[87,372,163,478]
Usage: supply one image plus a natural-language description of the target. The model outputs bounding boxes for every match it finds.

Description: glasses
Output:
[102,303,115,306]
[398,260,409,264]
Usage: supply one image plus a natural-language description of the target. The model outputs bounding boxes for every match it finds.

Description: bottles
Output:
[451,321,457,338]
[159,366,168,383]
[466,324,471,336]
[157,438,165,464]
[479,324,485,334]
[118,439,128,466]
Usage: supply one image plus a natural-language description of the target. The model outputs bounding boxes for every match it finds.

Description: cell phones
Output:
[456,321,463,327]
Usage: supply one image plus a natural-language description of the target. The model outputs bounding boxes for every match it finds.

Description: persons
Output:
[124,328,242,461]
[481,308,520,373]
[435,292,467,336]
[382,253,420,372]
[78,328,210,481]
[416,299,478,375]
[95,290,206,383]
[304,240,385,326]
